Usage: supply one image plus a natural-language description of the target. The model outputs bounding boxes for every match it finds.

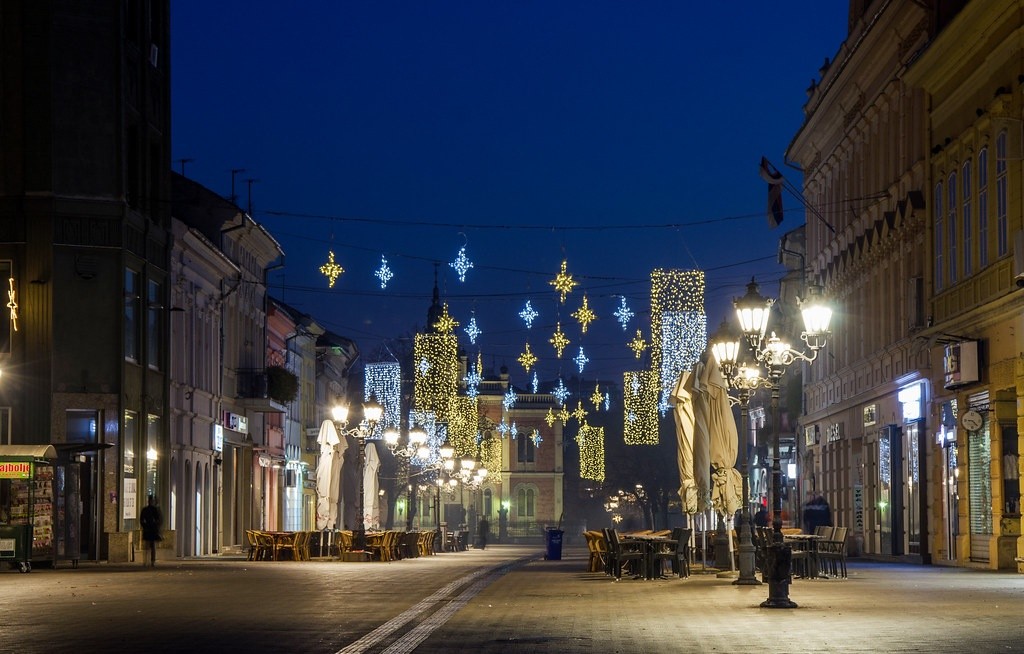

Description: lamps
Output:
[160,306,186,312]
[911,336,960,356]
[926,331,981,349]
[214,459,222,464]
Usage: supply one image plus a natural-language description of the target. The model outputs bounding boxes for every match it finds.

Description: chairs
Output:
[244,528,469,561]
[727,524,849,581]
[582,526,693,578]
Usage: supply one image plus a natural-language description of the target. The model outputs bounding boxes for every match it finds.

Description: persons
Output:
[140,499,162,566]
[479,515,490,549]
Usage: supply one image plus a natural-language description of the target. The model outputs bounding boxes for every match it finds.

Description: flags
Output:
[761,160,784,227]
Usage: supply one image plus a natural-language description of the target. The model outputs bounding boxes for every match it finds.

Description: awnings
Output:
[0,443,58,572]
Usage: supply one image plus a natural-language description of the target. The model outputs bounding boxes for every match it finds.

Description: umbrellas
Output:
[363,443,380,530]
[317,420,340,557]
[326,426,347,557]
[669,357,741,572]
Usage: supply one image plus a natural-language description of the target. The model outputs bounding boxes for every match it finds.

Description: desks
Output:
[622,537,679,579]
[785,534,826,579]
[261,532,291,561]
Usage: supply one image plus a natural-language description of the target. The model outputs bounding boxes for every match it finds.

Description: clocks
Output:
[961,410,984,432]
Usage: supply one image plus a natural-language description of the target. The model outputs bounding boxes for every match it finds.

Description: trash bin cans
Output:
[544,529,565,560]
